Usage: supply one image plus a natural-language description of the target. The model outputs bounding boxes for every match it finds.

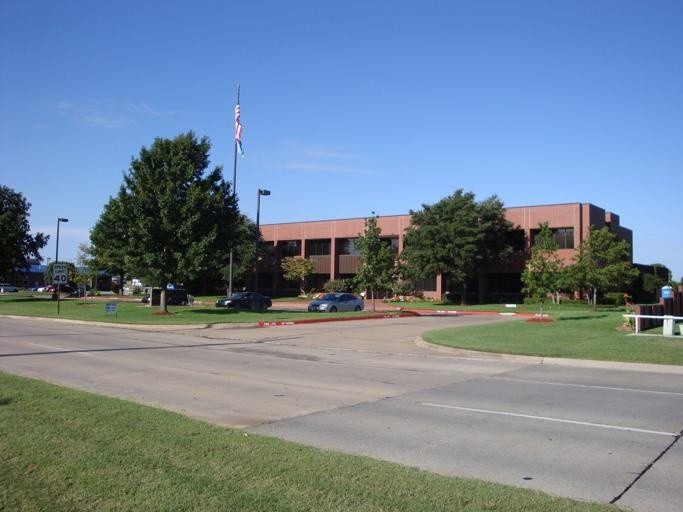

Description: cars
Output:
[215,292,271,309]
[307,292,363,312]
[0,283,18,292]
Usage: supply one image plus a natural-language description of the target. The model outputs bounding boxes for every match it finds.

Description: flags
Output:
[234,103,246,160]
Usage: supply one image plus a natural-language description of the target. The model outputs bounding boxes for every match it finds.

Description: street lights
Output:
[52,217,68,301]
[253,188,270,289]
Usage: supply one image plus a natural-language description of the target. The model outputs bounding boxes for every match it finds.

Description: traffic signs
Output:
[52,263,67,284]
